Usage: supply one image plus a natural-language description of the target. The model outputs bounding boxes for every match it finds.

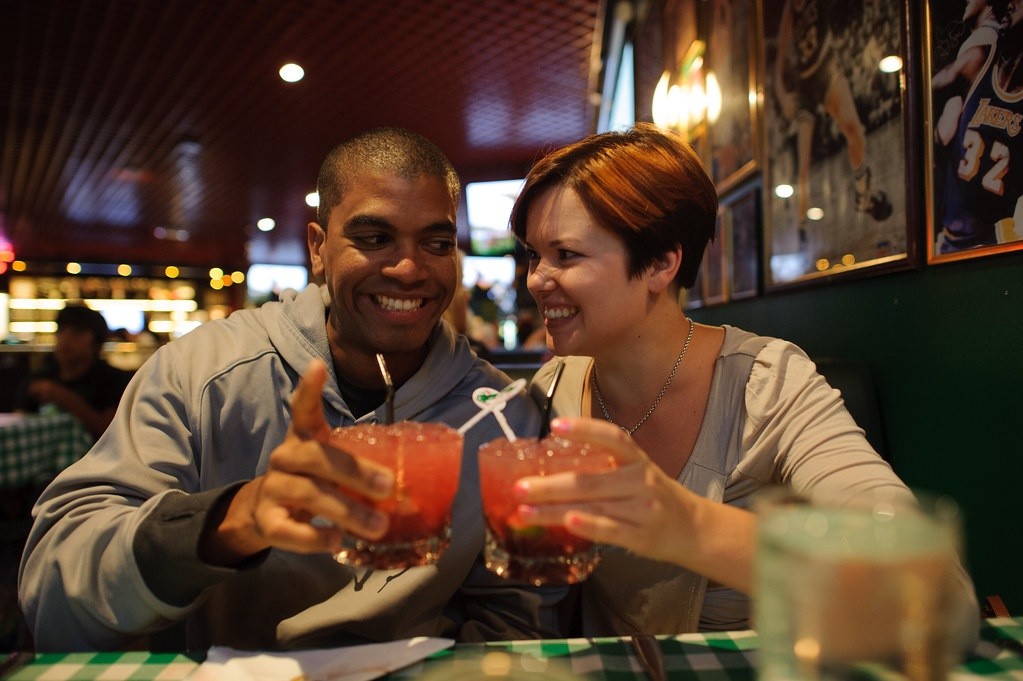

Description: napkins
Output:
[192,637,456,681]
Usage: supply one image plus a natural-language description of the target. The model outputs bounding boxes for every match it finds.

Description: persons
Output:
[765,0,901,245]
[929,0,1023,256]
[528,126,981,664]
[0,305,128,439]
[15,129,570,651]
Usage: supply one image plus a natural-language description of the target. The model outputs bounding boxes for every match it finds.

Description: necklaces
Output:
[591,317,693,435]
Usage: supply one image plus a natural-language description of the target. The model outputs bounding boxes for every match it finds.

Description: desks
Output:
[0,617,1023,681]
[0,412,93,484]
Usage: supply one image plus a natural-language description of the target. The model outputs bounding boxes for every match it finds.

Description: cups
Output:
[755,502,957,681]
[479,443,612,587]
[329,433,466,571]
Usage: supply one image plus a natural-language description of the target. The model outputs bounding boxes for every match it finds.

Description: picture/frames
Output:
[659,0,761,311]
[919,0,1022,266]
[755,0,919,294]
